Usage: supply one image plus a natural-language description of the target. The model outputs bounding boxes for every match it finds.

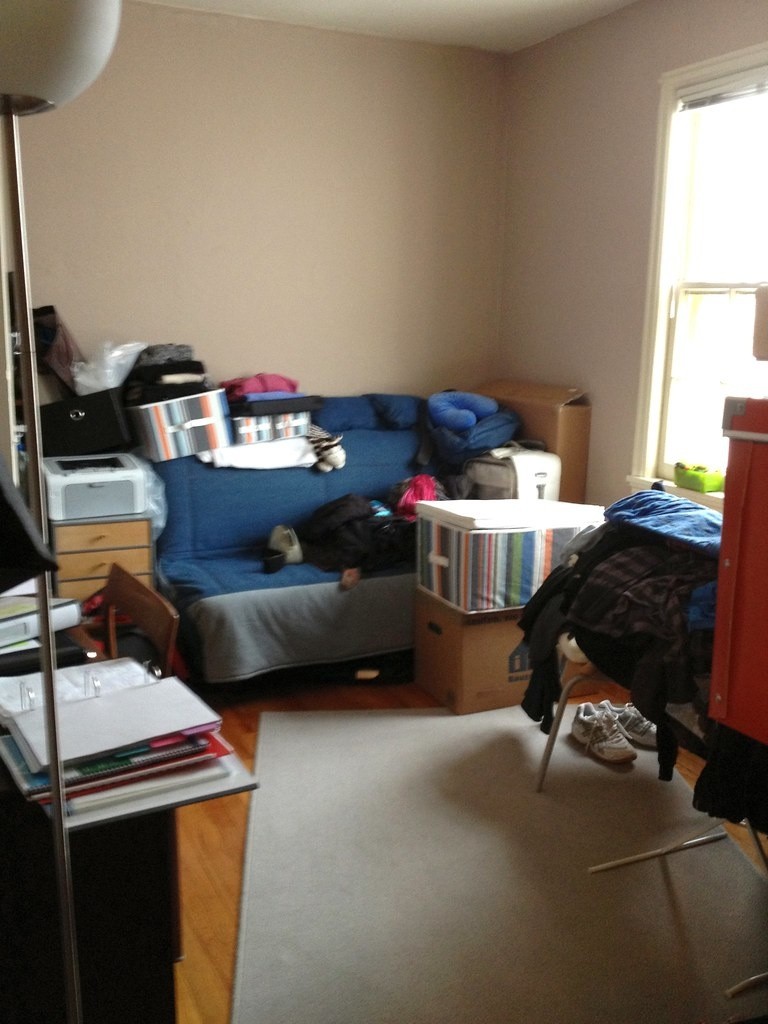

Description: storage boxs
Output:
[230,412,312,445]
[127,389,234,462]
[414,380,606,715]
[710,396,767,745]
[40,387,133,458]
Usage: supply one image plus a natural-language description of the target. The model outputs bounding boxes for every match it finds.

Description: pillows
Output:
[312,392,498,431]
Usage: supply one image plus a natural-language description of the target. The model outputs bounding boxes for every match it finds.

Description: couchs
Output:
[151,425,452,684]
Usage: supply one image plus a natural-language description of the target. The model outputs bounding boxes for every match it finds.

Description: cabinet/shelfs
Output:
[49,510,157,601]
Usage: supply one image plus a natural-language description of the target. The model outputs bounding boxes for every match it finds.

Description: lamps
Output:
[0,0,122,116]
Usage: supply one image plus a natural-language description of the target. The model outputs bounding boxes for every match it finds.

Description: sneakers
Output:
[574,698,659,766]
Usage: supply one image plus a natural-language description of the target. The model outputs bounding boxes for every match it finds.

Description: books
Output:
[0,591,235,818]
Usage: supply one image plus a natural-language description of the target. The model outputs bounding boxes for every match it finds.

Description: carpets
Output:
[230,703,768,1024]
